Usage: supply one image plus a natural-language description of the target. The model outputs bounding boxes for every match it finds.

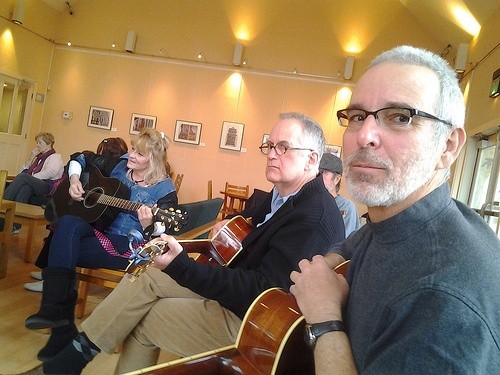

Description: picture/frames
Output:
[325,144,342,159]
[129,113,157,135]
[173,120,202,145]
[87,106,114,130]
[262,134,269,144]
[219,121,245,151]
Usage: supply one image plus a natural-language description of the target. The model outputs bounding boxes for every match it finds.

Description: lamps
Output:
[455,42,468,72]
[125,31,136,53]
[12,0,26,25]
[233,44,244,66]
[344,56,355,80]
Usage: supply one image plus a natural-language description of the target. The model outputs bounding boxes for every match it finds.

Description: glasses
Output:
[336,107,452,129]
[259,141,314,156]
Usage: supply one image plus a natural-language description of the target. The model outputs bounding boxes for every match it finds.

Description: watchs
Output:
[301,316,349,352]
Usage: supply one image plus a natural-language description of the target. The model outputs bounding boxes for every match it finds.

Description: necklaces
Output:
[130,169,145,185]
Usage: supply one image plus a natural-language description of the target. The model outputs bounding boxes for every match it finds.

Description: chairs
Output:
[76,174,249,318]
[0,202,15,278]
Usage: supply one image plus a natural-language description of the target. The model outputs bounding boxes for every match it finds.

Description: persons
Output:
[24,137,128,292]
[25,126,178,361]
[318,152,360,240]
[0,131,65,234]
[17,112,346,375]
[288,43,500,375]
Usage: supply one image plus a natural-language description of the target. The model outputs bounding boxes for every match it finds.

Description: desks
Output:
[220,192,250,214]
[0,199,50,263]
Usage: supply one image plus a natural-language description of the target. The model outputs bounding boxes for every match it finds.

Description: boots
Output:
[37,289,77,362]
[25,266,75,330]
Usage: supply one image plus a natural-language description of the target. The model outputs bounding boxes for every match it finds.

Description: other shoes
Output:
[24,271,43,291]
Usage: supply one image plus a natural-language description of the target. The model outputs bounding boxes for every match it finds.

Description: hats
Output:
[319,153,343,175]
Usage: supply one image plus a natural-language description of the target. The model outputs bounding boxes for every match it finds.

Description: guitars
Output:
[43,163,192,232]
[124,214,253,283]
[120,258,351,375]
